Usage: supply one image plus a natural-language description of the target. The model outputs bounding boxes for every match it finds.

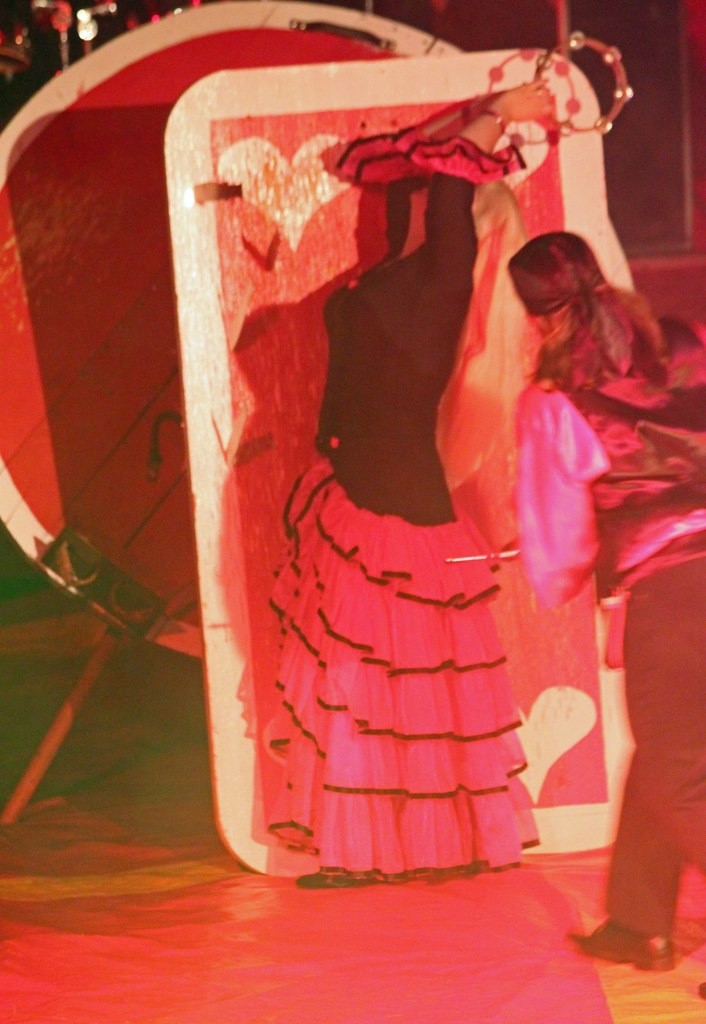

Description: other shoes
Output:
[296,866,374,888]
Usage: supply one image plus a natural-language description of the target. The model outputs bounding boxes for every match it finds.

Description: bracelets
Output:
[482,111,505,134]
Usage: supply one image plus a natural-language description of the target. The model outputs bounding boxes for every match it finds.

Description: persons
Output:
[506,231,706,1000]
[260,79,550,887]
[30,3,79,86]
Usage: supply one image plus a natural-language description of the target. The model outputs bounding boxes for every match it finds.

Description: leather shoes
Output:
[572,916,675,973]
